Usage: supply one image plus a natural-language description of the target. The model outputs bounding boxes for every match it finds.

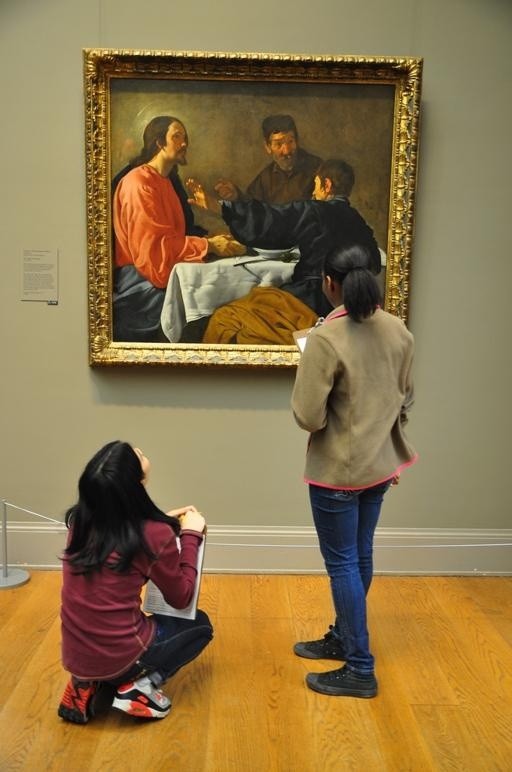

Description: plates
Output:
[252,245,295,261]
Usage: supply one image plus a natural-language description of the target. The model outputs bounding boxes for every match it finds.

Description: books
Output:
[142,533,206,622]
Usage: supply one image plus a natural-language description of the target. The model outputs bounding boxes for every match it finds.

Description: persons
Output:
[214,113,327,206]
[183,157,384,321]
[108,115,251,342]
[286,238,418,699]
[54,438,216,727]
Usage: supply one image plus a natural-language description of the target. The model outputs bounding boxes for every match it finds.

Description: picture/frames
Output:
[82,48,424,371]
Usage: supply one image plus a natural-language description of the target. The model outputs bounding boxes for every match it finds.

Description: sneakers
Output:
[57,674,104,725]
[293,630,346,662]
[111,681,173,723]
[306,664,378,699]
